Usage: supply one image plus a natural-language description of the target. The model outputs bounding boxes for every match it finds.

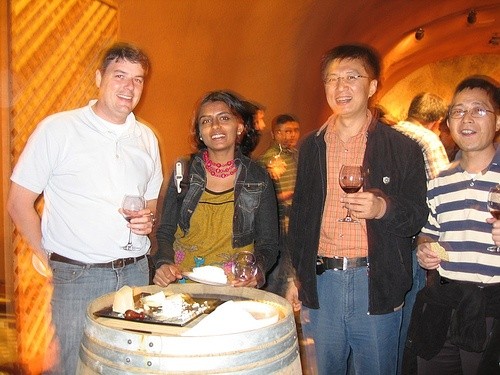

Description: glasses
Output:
[323,72,368,85]
[448,106,496,119]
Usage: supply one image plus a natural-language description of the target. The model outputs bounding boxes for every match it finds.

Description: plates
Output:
[93,292,220,328]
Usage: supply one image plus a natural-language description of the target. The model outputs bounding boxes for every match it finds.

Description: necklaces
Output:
[202,149,239,178]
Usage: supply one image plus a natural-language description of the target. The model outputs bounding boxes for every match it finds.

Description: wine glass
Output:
[339,166,363,223]
[487,185,500,252]
[231,251,256,280]
[123,194,144,251]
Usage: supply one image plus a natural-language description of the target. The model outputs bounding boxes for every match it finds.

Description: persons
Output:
[369,104,450,374]
[4,42,163,375]
[150,89,280,289]
[246,100,268,160]
[256,113,303,312]
[288,40,429,375]
[403,74,500,374]
[404,91,448,137]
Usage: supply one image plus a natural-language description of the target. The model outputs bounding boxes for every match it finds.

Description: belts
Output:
[316,257,368,270]
[50,252,145,268]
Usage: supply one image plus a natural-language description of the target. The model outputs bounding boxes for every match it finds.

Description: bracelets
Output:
[150,210,156,228]
